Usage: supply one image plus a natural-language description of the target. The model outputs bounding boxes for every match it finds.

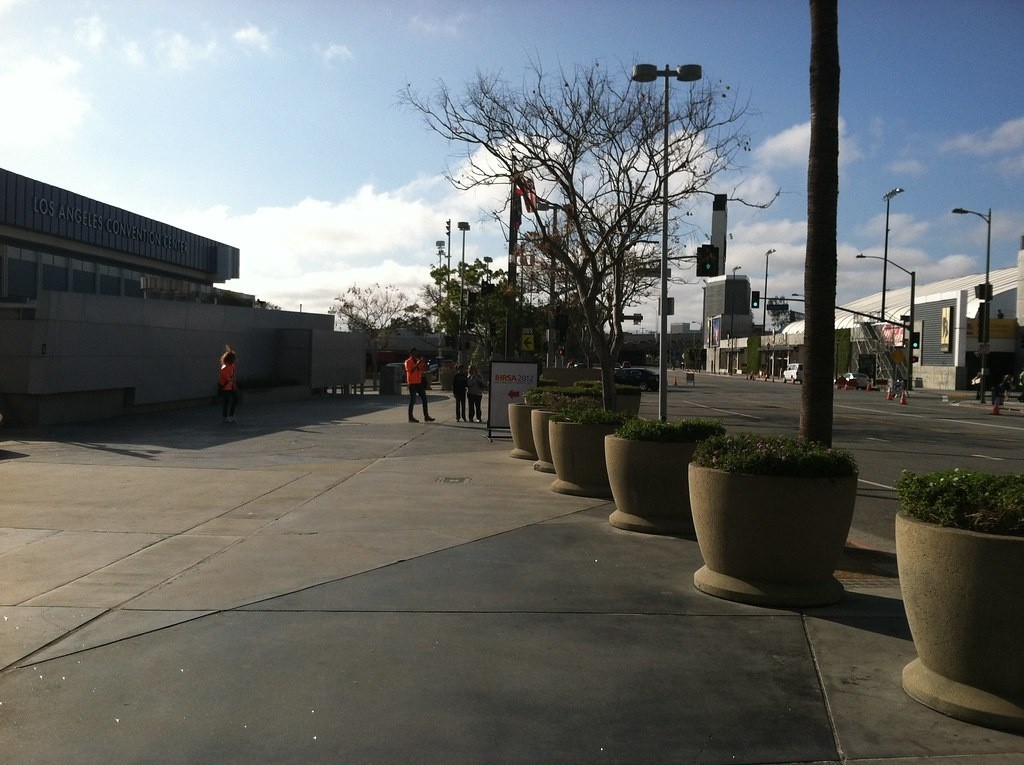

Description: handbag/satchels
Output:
[422,373,431,390]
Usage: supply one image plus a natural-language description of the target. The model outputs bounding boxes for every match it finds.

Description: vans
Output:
[782,363,804,384]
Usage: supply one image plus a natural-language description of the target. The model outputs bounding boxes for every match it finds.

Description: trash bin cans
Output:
[379,365,402,396]
[424,372,432,390]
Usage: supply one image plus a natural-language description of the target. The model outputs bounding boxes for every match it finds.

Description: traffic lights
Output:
[752,291,759,308]
[702,244,714,277]
[913,332,920,349]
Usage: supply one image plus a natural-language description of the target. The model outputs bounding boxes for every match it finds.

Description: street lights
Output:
[484,257,493,282]
[952,205,992,402]
[436,222,470,372]
[536,203,569,368]
[857,253,916,391]
[762,249,776,336]
[631,63,703,422]
[882,186,905,319]
[730,266,741,374]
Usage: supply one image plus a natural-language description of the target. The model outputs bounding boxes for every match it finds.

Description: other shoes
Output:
[224,415,238,423]
[424,416,435,422]
[409,417,419,422]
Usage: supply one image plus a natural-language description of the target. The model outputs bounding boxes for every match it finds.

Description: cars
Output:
[837,373,870,389]
[615,367,659,392]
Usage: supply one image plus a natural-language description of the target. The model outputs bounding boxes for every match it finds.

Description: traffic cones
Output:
[866,382,872,391]
[844,384,849,391]
[887,387,907,404]
[988,400,1002,416]
[672,377,678,386]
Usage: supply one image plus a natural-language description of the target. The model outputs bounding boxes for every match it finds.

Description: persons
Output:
[453,364,485,423]
[997,309,1005,319]
[219,345,240,424]
[971,371,1024,403]
[404,347,436,423]
